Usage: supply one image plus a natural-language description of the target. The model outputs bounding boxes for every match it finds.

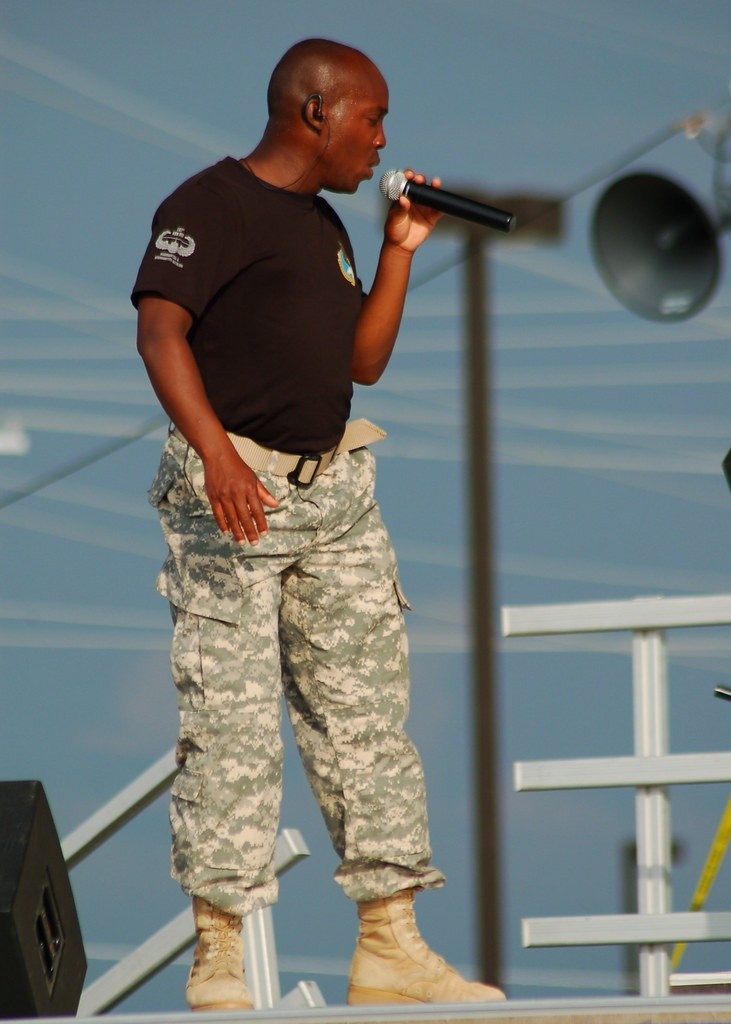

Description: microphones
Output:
[379,170,517,232]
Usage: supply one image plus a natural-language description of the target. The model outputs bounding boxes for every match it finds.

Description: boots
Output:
[348,889,507,1005]
[185,895,256,1011]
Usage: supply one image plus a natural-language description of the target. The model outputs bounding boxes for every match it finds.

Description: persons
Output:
[126,36,513,1011]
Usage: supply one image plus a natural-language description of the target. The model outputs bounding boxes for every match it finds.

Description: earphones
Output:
[303,94,323,121]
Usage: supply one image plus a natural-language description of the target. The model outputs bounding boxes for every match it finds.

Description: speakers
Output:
[0,780,88,1016]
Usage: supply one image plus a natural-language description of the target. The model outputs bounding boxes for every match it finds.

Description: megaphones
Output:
[594,169,731,322]
